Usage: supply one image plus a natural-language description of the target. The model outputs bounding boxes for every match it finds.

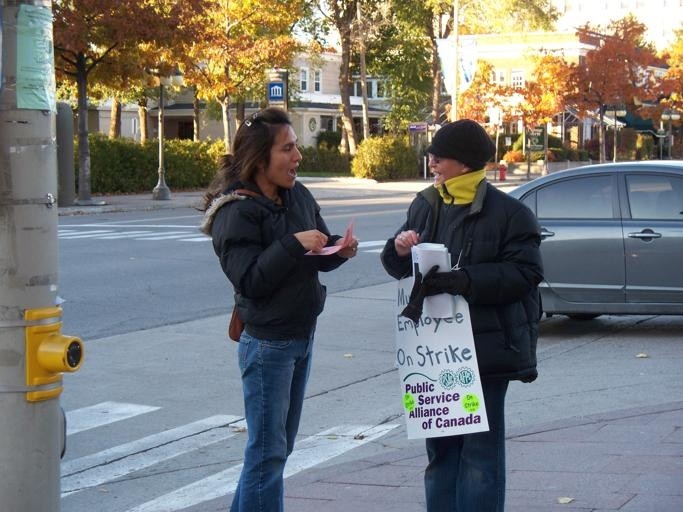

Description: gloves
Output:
[401,266,439,324]
[424,269,470,297]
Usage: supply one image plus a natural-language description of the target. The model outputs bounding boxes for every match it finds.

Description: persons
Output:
[196,105,356,511]
[379,119,544,511]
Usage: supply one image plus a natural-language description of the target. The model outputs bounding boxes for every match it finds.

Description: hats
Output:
[425,119,496,170]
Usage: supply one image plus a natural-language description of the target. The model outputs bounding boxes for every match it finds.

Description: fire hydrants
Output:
[497,164,508,181]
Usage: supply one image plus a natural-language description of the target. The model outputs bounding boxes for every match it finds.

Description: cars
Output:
[505,159,682,324]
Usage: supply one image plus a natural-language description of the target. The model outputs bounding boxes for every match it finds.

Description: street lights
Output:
[142,62,184,201]
[660,107,680,160]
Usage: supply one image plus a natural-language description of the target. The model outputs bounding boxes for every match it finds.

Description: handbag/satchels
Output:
[229,303,244,342]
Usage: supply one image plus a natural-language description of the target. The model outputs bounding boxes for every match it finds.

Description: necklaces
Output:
[274,197,278,202]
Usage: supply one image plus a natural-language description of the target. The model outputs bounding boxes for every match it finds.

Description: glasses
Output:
[428,152,443,163]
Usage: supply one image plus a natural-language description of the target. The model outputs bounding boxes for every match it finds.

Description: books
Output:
[407,240,453,318]
